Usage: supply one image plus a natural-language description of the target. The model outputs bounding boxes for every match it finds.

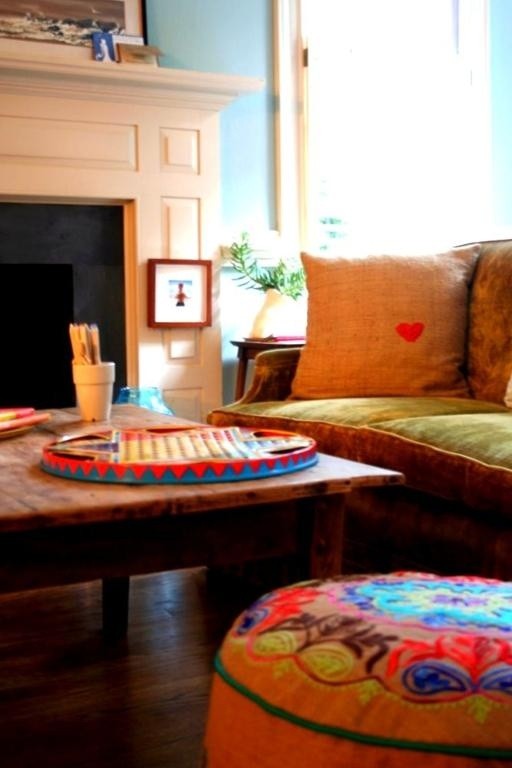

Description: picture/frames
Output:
[0,0,149,60]
[148,259,213,327]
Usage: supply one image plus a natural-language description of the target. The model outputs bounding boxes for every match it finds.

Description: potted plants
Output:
[229,218,342,341]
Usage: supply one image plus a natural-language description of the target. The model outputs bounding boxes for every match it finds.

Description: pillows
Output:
[285,252,479,399]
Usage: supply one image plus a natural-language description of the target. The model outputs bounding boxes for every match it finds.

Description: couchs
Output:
[206,239,512,583]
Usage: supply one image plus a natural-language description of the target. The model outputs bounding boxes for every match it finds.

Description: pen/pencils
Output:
[69,322,101,366]
[0,407,51,431]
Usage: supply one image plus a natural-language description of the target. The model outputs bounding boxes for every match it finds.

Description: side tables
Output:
[231,341,305,401]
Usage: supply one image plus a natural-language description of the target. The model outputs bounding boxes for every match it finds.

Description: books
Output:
[48,430,120,461]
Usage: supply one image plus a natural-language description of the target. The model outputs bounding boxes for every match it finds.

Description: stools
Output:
[204,569,512,768]
